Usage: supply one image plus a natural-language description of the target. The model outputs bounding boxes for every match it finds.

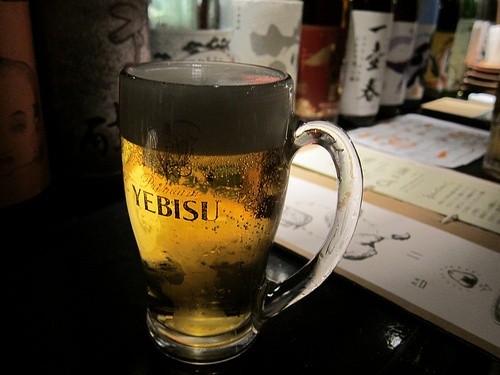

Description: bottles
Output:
[0,0,500,230]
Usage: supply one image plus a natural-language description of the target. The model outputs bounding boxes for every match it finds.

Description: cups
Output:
[118,60,364,367]
